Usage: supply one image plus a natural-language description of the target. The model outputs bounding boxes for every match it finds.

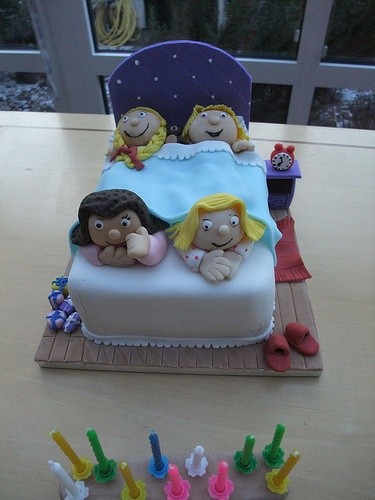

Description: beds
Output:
[67,41,283,349]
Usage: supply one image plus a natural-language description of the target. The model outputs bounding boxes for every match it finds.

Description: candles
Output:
[46,460,80,498]
[241,434,255,466]
[118,460,139,498]
[47,431,85,472]
[148,432,164,471]
[272,450,301,486]
[192,445,205,469]
[86,427,110,473]
[214,461,228,492]
[268,422,285,459]
[168,464,182,496]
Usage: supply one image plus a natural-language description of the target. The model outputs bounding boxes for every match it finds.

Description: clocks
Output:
[272,142,295,171]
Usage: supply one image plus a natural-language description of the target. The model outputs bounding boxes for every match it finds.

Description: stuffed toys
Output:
[111,107,167,171]
[165,194,268,282]
[69,188,171,267]
[167,105,256,154]
